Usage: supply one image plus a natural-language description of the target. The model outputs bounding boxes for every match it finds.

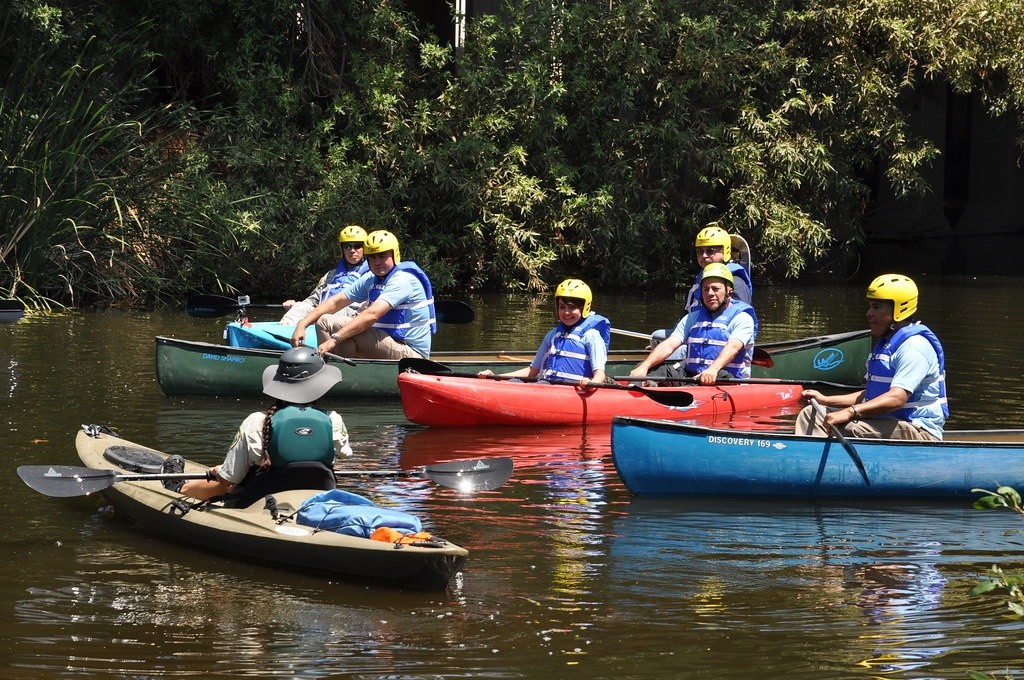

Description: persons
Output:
[280,226,370,324]
[478,279,608,392]
[629,263,757,386]
[795,273,949,440]
[645,227,752,360]
[161,346,352,501]
[291,230,437,359]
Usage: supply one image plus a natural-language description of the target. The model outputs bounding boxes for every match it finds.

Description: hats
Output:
[262,346,341,404]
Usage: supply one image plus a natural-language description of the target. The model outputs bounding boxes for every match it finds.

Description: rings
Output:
[318,351,320,353]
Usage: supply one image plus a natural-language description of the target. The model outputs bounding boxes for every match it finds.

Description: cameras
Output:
[238,295,250,305]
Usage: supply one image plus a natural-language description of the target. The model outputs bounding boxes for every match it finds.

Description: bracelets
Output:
[332,333,341,343]
[850,404,861,424]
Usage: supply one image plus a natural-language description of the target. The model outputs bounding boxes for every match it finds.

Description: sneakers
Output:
[162,455,185,492]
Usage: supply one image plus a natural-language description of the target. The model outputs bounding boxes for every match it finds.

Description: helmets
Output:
[363,229,400,264]
[555,278,593,318]
[700,261,734,289]
[695,227,731,262]
[866,273,918,321]
[337,226,367,254]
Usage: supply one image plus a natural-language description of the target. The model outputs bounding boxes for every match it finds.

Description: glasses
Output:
[696,248,721,257]
[340,244,362,250]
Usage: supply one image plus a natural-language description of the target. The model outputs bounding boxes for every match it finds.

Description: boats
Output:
[224,321,319,352]
[611,414,1024,500]
[75,423,470,593]
[396,368,808,427]
[153,329,876,398]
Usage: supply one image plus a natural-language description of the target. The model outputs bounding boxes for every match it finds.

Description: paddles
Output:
[16,456,516,499]
[806,395,871,486]
[185,293,477,325]
[751,346,775,369]
[262,329,358,368]
[610,374,867,391]
[397,357,695,407]
